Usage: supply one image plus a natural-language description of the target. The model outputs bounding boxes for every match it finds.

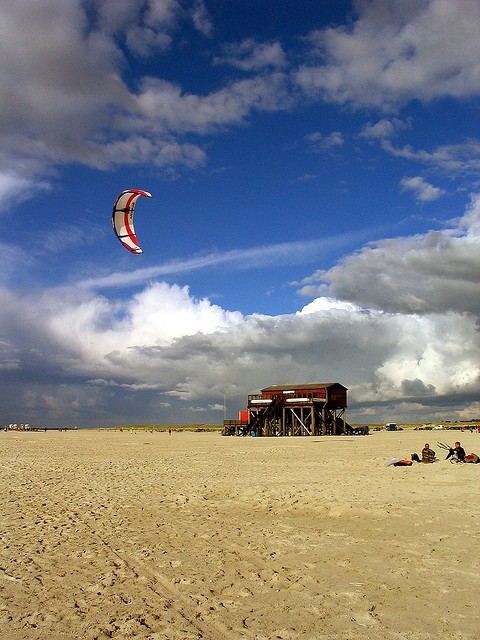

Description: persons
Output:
[421,443,436,463]
[443,441,466,463]
